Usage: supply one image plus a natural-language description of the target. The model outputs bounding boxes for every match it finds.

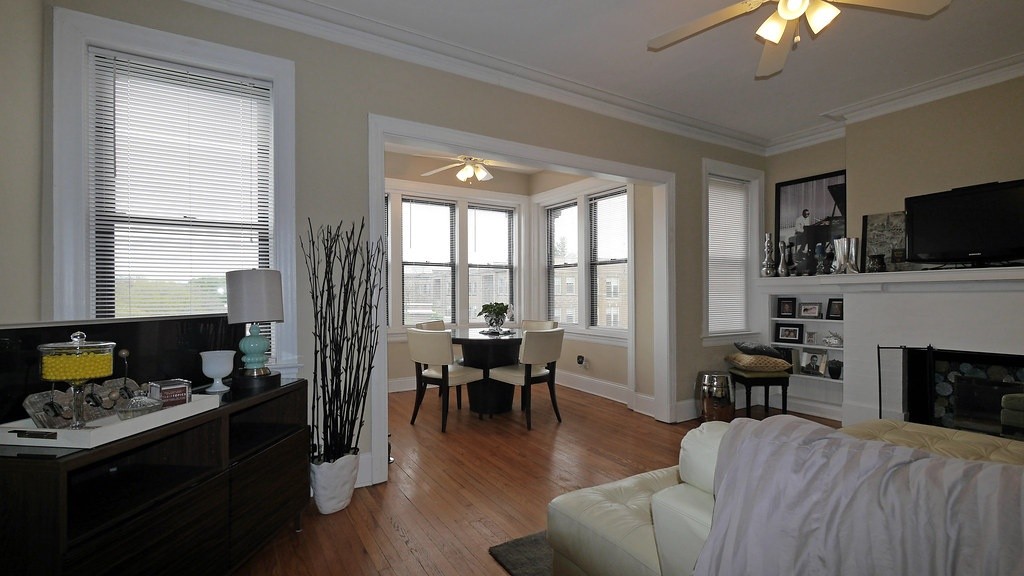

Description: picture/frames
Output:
[775,322,804,343]
[799,302,822,319]
[774,170,846,271]
[776,297,796,318]
[800,348,828,376]
[825,297,843,320]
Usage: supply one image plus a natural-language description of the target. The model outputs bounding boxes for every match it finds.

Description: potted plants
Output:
[476,302,510,333]
[826,359,843,379]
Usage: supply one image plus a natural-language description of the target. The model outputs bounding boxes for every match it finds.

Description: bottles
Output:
[867,254,887,273]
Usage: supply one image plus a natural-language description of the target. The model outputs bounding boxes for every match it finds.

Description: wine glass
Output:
[200,350,236,391]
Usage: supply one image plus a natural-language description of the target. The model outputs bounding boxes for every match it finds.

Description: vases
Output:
[310,446,361,515]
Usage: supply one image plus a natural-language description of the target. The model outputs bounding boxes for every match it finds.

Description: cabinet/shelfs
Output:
[0,377,311,576]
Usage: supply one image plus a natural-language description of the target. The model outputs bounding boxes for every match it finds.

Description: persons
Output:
[806,355,819,371]
[810,307,816,314]
[789,331,794,337]
[795,209,810,253]
[784,330,789,337]
[835,305,840,314]
[783,304,790,313]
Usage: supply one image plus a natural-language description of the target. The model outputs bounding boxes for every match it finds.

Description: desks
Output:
[451,324,524,416]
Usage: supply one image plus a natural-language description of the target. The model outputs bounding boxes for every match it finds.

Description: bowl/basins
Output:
[114,389,163,421]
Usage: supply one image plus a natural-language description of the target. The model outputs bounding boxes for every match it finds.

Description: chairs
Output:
[417,321,459,398]
[405,327,484,433]
[488,327,566,431]
[519,317,558,366]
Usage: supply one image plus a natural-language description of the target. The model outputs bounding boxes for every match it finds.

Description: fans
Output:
[646,0,953,80]
[414,152,522,178]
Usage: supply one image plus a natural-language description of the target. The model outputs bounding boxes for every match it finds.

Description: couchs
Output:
[544,415,1023,576]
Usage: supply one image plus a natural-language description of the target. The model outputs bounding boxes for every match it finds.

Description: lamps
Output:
[756,0,842,45]
[455,160,494,184]
[225,268,284,377]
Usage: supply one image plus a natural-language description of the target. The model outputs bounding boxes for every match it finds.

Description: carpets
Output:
[489,527,554,576]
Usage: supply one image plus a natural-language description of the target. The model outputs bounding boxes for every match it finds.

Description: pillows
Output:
[724,341,793,372]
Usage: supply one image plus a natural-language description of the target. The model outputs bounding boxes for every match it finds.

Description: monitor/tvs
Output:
[905,178,1024,270]
